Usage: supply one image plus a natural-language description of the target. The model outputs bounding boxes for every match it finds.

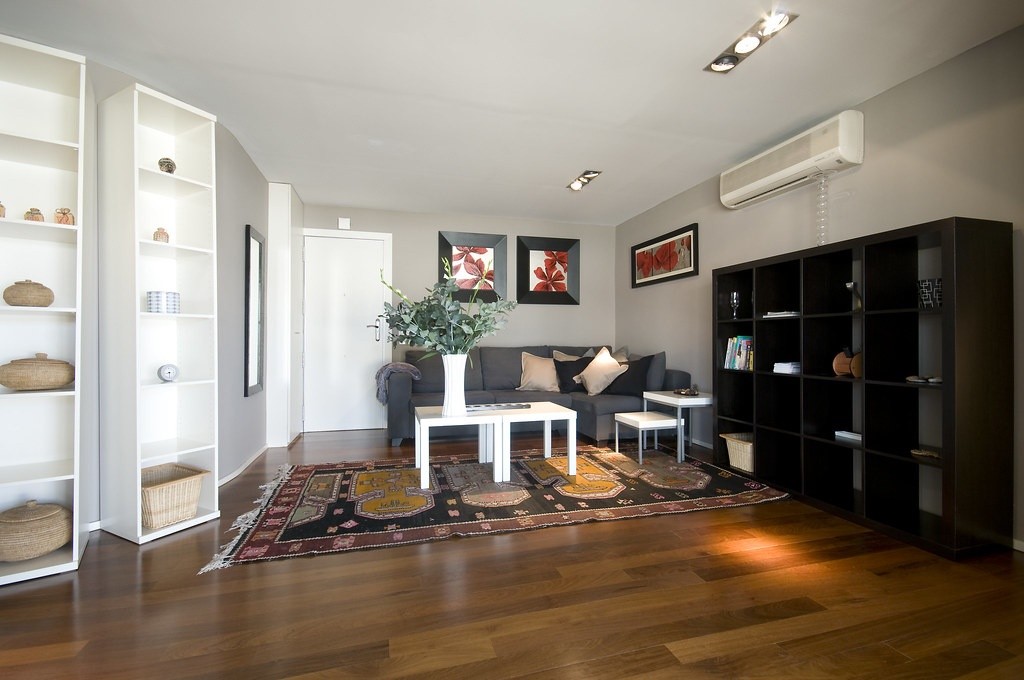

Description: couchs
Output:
[572,367,691,448]
[385,345,612,445]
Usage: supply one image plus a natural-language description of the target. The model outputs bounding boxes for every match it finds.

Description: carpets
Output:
[196,436,791,573]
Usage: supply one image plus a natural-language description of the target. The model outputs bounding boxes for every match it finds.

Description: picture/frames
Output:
[437,230,508,304]
[517,236,581,305]
[631,222,699,289]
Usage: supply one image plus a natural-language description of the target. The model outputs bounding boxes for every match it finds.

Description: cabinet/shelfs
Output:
[709,216,1013,561]
[1,34,90,585]
[97,82,226,543]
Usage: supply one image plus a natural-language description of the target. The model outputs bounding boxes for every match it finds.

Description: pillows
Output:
[514,344,666,399]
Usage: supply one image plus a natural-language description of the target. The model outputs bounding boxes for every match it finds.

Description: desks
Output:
[642,389,714,462]
[413,400,578,490]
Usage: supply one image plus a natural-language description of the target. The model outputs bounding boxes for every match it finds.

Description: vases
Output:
[441,353,468,418]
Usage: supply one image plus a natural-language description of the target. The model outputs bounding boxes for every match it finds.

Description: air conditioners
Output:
[719,110,864,210]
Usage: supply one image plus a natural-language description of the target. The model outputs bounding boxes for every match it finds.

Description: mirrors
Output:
[244,223,263,396]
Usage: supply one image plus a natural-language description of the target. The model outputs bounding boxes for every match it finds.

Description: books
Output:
[773,362,800,374]
[762,311,800,318]
[724,335,754,371]
[834,430,861,440]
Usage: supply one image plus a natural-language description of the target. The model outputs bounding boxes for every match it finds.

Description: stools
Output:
[614,411,685,466]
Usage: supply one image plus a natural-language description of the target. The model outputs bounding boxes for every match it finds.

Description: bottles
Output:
[153,228,168,242]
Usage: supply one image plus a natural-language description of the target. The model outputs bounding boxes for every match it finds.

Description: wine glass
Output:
[730,292,739,319]
